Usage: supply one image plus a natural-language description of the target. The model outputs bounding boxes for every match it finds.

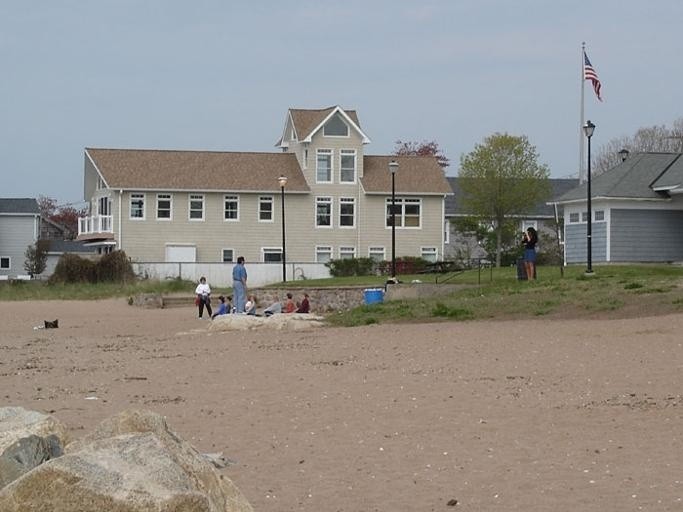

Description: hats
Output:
[527,227,535,232]
[247,294,257,301]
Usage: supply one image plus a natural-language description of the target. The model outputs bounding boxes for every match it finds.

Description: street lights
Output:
[582,120,596,278]
[277,174,289,283]
[386,160,400,285]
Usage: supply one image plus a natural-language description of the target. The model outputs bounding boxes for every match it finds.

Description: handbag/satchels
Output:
[195,295,201,304]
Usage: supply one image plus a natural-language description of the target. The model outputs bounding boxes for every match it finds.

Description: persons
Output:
[231,257,249,315]
[194,277,213,319]
[522,227,538,281]
[211,293,310,320]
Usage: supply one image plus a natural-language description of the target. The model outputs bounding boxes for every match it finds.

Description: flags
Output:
[584,52,602,102]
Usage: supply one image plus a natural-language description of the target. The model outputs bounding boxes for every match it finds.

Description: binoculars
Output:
[522,231,530,234]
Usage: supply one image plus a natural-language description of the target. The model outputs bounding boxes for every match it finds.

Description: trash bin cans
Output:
[502,255,512,266]
[517,258,536,279]
[365,288,384,303]
